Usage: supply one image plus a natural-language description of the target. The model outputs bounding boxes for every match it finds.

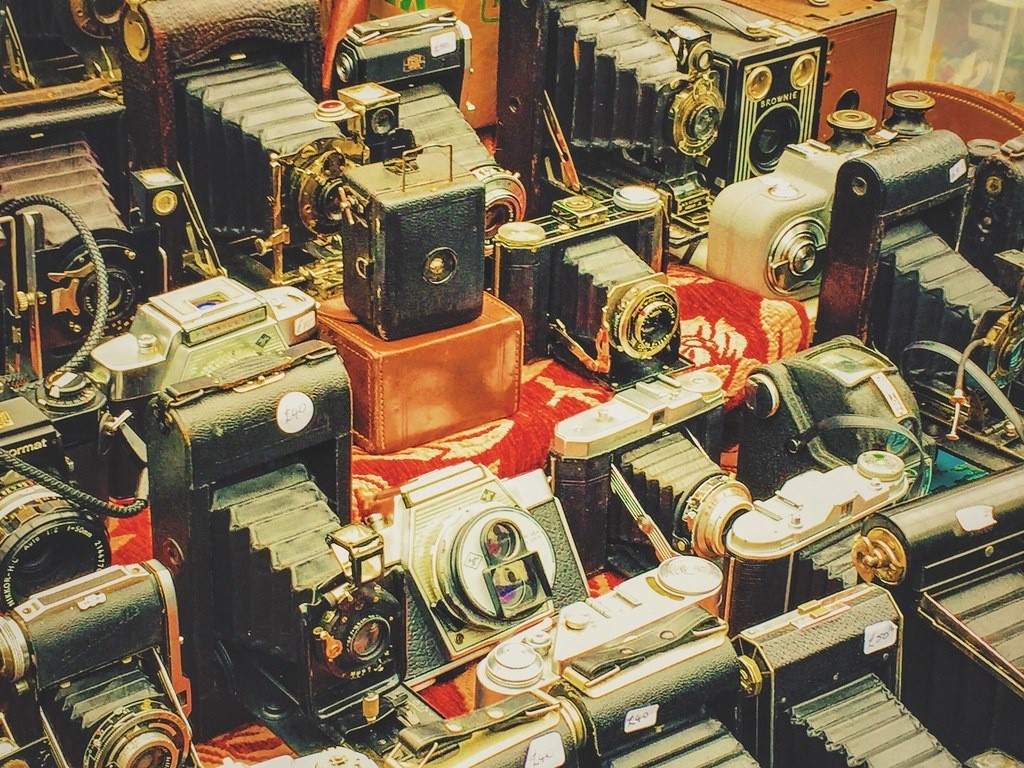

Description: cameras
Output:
[1,0,1024,768]
